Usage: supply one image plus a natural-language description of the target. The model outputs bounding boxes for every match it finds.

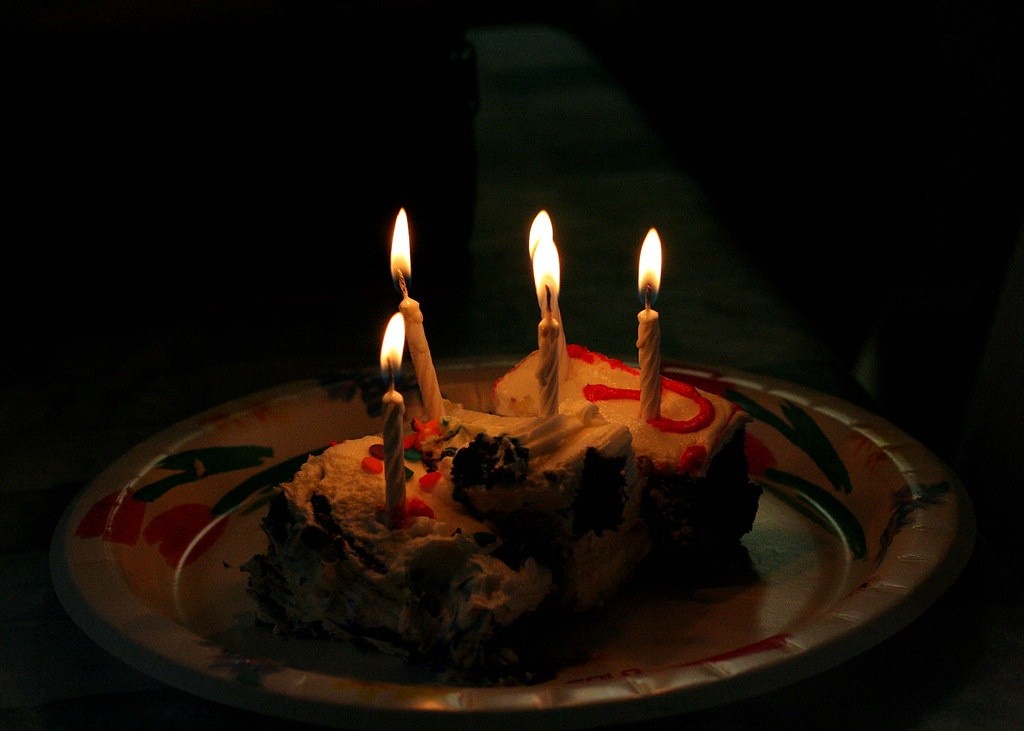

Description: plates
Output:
[48,353,977,731]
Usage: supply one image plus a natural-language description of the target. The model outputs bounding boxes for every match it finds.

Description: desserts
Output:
[240,340,760,678]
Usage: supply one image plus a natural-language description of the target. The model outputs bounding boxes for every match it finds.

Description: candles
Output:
[636,228,664,421]
[379,311,407,519]
[532,237,561,416]
[389,207,445,420]
[528,210,574,382]
